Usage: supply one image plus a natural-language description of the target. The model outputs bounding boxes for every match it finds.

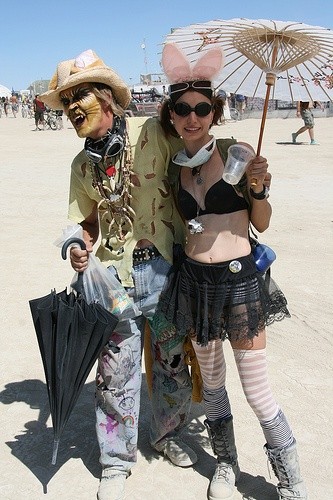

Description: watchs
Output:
[248,184,270,200]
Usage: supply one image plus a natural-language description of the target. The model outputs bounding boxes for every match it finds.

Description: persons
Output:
[156,43,308,500]
[37,49,272,500]
[0,92,51,132]
[215,89,248,124]
[292,101,325,145]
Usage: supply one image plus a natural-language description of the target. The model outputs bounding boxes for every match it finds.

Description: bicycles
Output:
[28,105,34,118]
[37,109,62,130]
[22,105,30,118]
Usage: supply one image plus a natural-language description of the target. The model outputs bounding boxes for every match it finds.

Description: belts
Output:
[133,246,161,261]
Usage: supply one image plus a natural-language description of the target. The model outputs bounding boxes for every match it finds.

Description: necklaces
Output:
[194,164,205,185]
[86,125,138,243]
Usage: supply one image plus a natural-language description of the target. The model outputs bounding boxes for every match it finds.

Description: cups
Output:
[222,144,257,186]
[253,244,276,273]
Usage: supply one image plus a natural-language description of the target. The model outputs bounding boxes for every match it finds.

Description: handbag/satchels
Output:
[55,222,143,323]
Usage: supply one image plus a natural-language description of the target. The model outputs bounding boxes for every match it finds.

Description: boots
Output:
[263,437,307,500]
[204,415,241,500]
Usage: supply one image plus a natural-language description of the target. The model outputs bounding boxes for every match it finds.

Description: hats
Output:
[36,49,131,110]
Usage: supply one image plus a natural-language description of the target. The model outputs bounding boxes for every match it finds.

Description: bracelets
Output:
[297,111,301,112]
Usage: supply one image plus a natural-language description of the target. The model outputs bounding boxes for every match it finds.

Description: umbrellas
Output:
[29,238,119,465]
[162,18,333,156]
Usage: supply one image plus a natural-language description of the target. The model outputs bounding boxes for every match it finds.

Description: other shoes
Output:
[292,133,296,143]
[310,141,320,145]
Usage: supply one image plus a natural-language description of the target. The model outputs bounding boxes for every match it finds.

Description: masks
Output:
[172,141,216,169]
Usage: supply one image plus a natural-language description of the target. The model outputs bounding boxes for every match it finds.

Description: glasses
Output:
[171,102,215,117]
[85,134,124,164]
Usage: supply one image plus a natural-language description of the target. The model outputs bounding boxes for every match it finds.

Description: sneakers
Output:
[161,439,198,467]
[97,470,126,500]
